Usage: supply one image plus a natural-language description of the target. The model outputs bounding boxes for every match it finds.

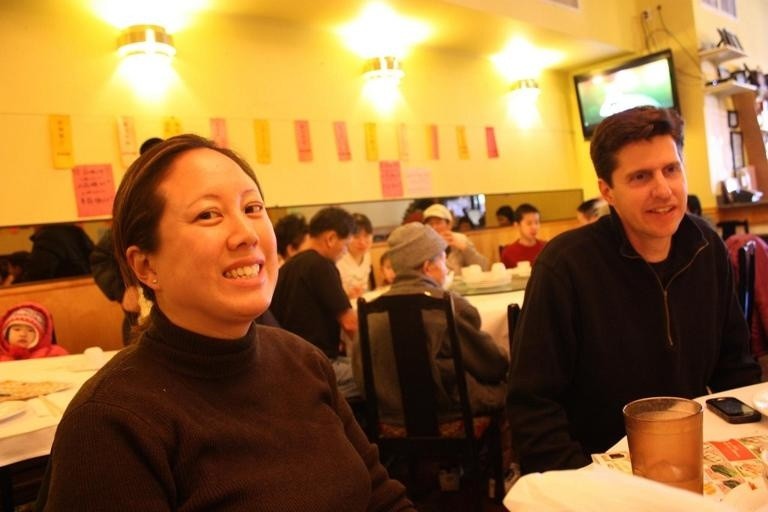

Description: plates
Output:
[752,392,768,416]
[0,399,26,422]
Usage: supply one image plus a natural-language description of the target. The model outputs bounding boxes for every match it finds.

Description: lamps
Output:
[113,26,178,107]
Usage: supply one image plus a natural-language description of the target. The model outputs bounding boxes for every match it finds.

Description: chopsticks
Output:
[37,393,64,426]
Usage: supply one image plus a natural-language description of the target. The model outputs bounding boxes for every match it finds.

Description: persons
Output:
[501,204,548,268]
[422,203,489,276]
[0,301,68,362]
[7,249,30,285]
[334,212,373,313]
[273,212,309,269]
[270,207,361,399]
[506,105,763,475]
[496,203,515,228]
[575,198,599,227]
[379,250,397,284]
[42,133,420,512]
[87,137,164,347]
[351,220,515,485]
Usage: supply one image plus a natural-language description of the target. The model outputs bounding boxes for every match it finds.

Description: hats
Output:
[3,308,45,348]
[422,204,452,221]
[387,221,447,275]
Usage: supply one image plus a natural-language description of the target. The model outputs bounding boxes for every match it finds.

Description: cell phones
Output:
[706,396,762,424]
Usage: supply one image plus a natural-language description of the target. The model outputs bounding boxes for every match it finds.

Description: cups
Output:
[467,263,483,284]
[623,397,704,496]
[491,262,505,276]
[518,261,532,275]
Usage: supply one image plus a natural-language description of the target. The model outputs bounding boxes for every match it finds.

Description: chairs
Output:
[734,236,765,348]
[358,294,503,510]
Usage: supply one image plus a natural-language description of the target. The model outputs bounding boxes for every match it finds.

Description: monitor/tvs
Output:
[572,48,680,140]
